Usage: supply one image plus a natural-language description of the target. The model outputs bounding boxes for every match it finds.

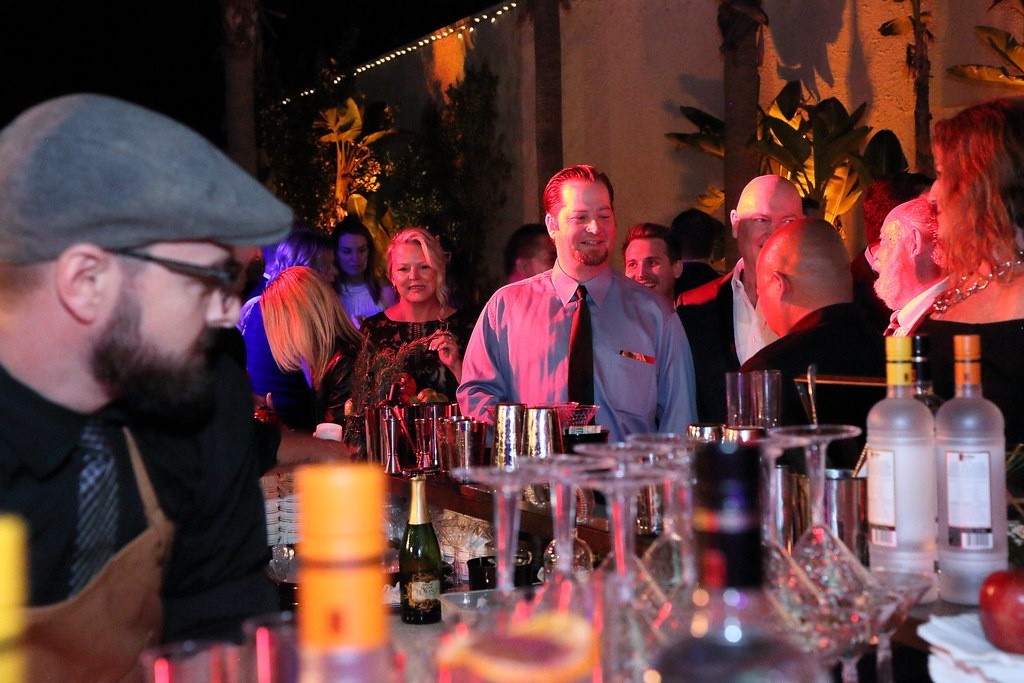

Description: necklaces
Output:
[932,243,1024,312]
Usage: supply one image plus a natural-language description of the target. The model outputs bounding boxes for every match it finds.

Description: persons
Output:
[357,227,477,406]
[456,163,699,445]
[202,95,1024,566]
[0,92,352,683]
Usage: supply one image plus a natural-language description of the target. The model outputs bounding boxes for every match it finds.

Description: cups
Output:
[750,367,783,425]
[725,371,751,426]
[465,553,532,592]
[364,404,601,503]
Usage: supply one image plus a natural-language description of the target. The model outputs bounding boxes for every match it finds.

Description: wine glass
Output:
[442,427,933,683]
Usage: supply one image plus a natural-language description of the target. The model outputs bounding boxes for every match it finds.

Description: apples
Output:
[979,570,1024,654]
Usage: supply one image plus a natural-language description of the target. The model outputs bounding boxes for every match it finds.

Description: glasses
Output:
[114,251,242,292]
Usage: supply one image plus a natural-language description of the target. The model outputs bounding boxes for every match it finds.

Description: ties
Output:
[882,316,900,337]
[567,285,596,426]
[70,424,123,596]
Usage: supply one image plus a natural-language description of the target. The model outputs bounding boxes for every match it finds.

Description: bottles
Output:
[399,468,443,626]
[865,335,937,603]
[286,460,414,682]
[914,334,945,420]
[939,334,1008,605]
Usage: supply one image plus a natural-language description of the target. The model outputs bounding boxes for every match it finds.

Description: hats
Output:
[0,92,295,270]
